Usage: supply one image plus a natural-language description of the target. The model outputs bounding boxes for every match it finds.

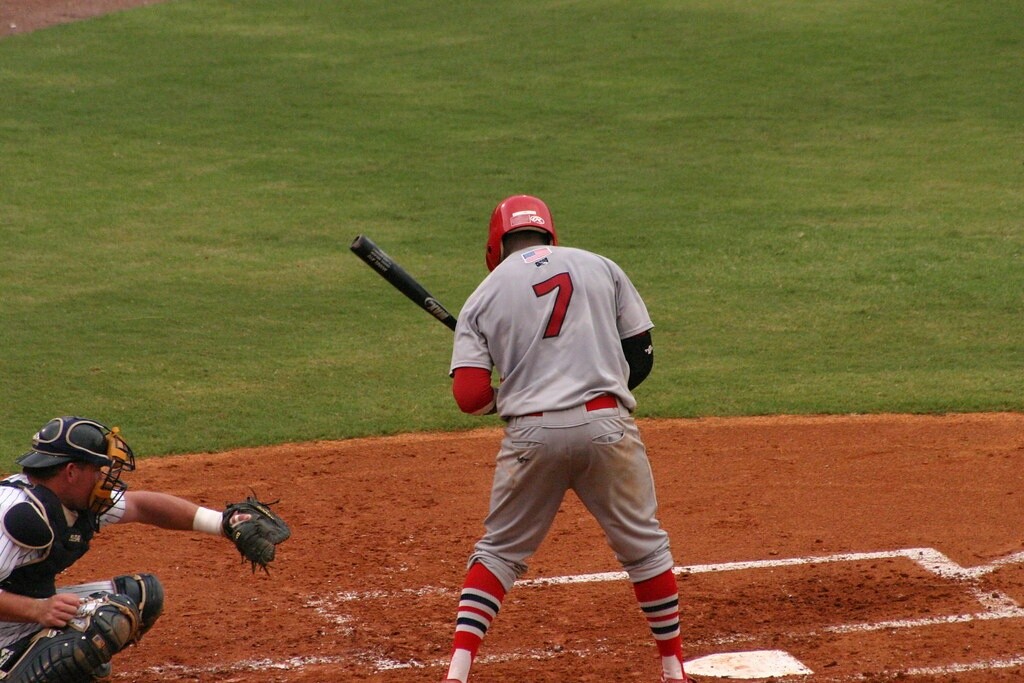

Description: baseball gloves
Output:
[222,500,292,568]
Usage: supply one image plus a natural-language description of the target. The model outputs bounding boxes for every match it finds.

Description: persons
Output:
[0,418,289,683]
[439,195,691,683]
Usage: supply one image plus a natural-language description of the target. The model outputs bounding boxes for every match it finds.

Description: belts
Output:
[525,394,619,416]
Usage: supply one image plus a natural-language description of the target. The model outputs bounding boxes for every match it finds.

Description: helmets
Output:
[14,415,112,468]
[485,195,559,271]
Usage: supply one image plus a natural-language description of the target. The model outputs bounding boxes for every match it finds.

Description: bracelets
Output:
[194,507,222,534]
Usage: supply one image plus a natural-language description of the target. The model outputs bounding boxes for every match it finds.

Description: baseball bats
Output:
[348,233,457,332]
